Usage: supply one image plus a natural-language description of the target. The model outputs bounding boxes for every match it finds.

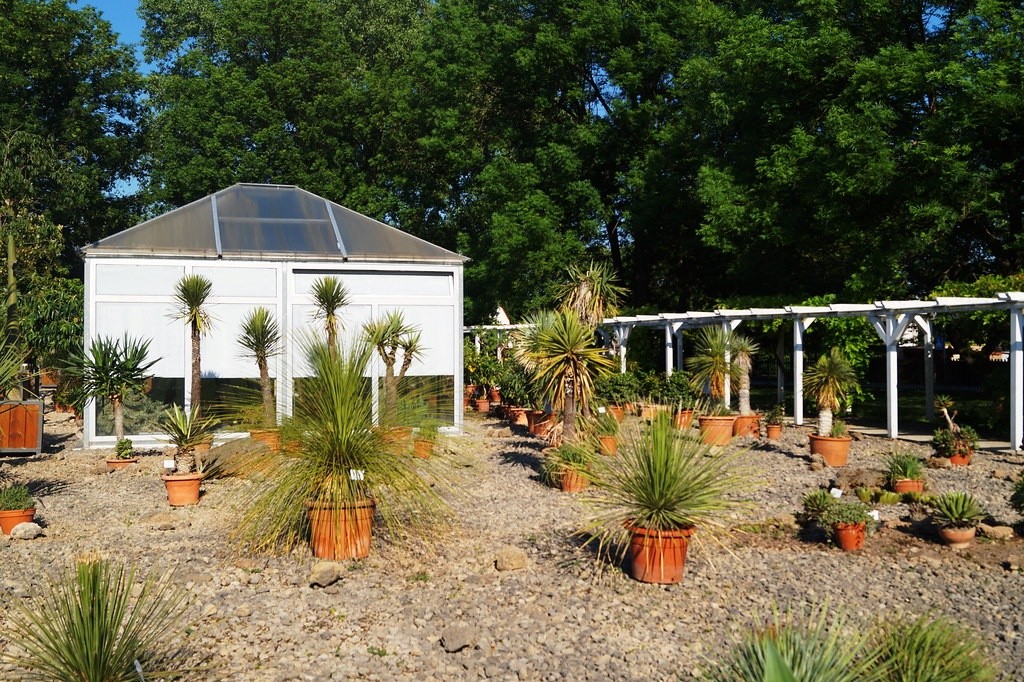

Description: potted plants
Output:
[823,501,874,553]
[55,328,164,471]
[280,415,303,455]
[799,346,864,468]
[412,418,443,460]
[765,405,784,438]
[71,386,84,421]
[358,306,434,456]
[929,493,982,542]
[886,454,925,497]
[0,481,39,534]
[468,261,763,587]
[55,393,65,413]
[189,325,478,561]
[65,394,75,414]
[162,273,226,458]
[931,394,981,465]
[235,306,283,456]
[153,401,219,505]
